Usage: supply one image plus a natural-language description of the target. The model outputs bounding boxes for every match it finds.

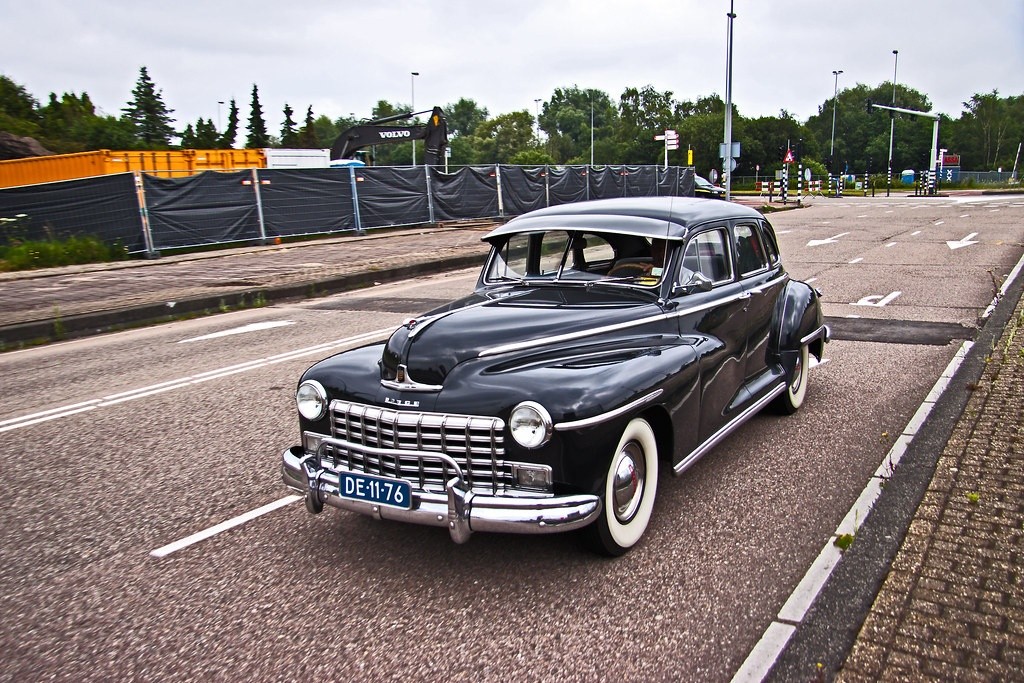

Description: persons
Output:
[634,238,695,292]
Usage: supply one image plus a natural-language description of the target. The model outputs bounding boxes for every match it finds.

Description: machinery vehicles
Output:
[263,106,451,170]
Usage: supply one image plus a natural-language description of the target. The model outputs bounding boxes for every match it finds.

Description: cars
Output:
[692,174,727,202]
[281,197,831,559]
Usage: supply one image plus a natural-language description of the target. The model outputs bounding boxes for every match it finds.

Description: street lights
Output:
[534,99,542,141]
[829,70,844,156]
[411,72,419,112]
[889,50,898,165]
